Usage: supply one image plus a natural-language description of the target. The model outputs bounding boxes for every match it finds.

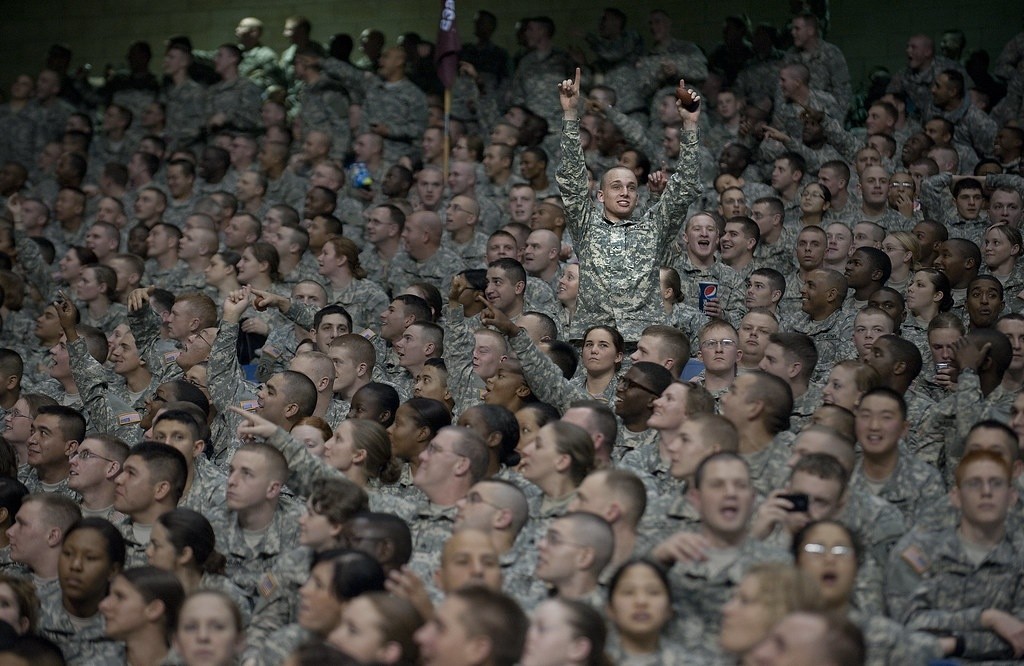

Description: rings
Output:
[716,308,718,313]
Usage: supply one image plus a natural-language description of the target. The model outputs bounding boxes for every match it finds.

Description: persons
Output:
[0,1,1023,665]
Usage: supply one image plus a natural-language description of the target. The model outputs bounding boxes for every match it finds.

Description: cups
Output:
[699,282,718,317]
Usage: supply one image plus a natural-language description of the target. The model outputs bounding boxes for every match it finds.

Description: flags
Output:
[432,0,462,87]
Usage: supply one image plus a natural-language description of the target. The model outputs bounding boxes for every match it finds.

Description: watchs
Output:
[959,366,978,375]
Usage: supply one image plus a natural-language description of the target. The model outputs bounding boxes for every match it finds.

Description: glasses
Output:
[181,377,207,389]
[881,247,906,251]
[889,182,914,188]
[804,543,852,557]
[463,494,513,528]
[701,339,738,348]
[617,375,661,398]
[546,535,583,548]
[447,204,474,215]
[335,535,383,547]
[195,331,212,348]
[69,449,113,463]
[6,408,32,419]
[427,443,465,458]
